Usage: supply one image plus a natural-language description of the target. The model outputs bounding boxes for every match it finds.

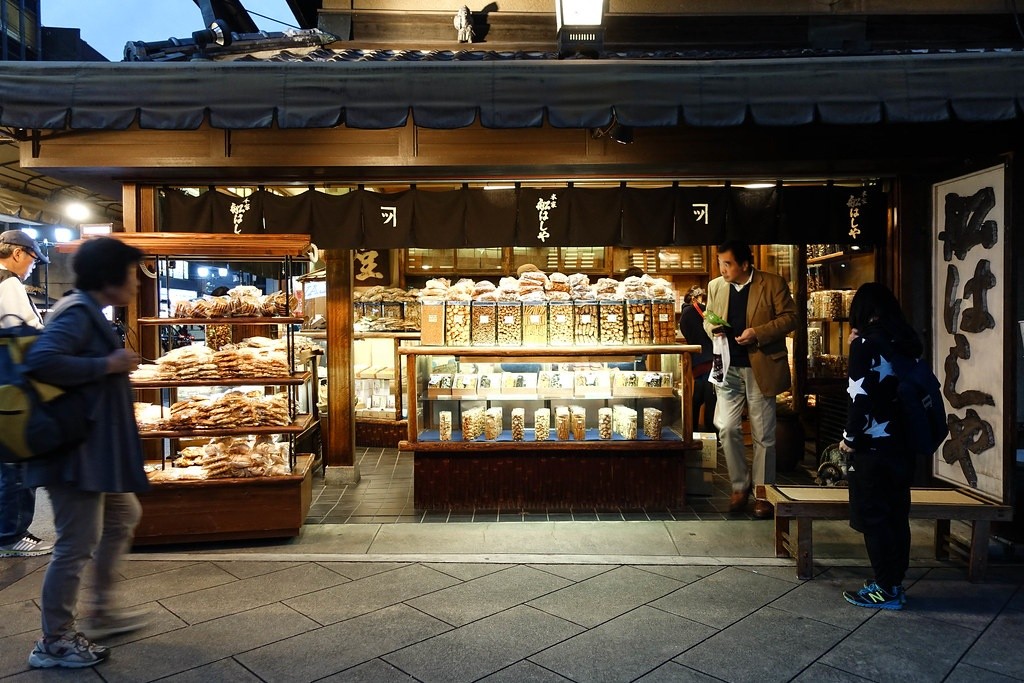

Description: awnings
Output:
[0,50,1024,180]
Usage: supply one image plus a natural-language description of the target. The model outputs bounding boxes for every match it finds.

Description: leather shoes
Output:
[755,500,773,517]
[730,480,753,511]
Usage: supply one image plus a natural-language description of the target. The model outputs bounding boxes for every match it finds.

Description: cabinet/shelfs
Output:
[808,250,854,412]
[399,344,707,514]
[304,332,420,450]
[129,235,316,542]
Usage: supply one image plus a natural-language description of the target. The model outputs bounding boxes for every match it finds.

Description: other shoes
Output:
[717,441,723,450]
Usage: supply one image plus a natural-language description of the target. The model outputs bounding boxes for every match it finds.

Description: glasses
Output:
[22,249,41,264]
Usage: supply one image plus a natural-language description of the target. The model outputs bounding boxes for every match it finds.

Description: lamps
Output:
[555,0,609,59]
[610,125,637,146]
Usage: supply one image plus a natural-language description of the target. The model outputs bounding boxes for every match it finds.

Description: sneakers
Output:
[76,610,150,641]
[0,531,55,557]
[842,578,906,610]
[29,630,109,669]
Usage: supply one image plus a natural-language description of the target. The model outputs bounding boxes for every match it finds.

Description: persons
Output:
[703,244,801,519]
[680,285,724,451]
[0,228,55,556]
[837,282,917,612]
[22,238,160,669]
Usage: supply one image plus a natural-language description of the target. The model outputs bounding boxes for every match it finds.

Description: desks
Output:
[751,483,1011,584]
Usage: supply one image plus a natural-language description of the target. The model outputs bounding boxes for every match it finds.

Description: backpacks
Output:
[0,293,121,463]
[855,332,947,459]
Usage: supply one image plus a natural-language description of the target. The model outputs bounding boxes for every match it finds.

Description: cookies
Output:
[175,293,298,317]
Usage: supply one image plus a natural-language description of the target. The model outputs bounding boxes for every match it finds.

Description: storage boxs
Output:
[420,295,677,350]
[354,298,419,336]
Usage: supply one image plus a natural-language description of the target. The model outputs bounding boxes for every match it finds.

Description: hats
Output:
[684,285,707,304]
[0,230,51,265]
